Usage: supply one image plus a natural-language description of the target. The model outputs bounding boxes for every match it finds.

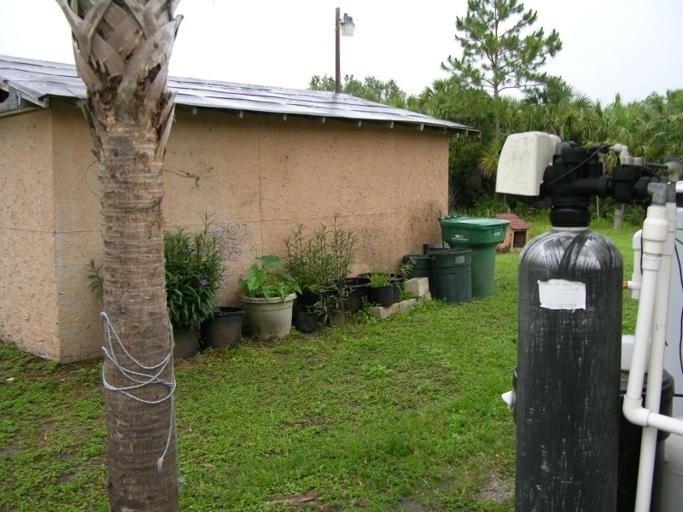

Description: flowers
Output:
[156,224,222,326]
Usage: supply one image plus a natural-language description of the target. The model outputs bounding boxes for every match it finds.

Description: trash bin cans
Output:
[437,214,511,298]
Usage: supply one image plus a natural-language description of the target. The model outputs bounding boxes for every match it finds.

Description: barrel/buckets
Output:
[402,252,428,278]
[430,248,474,303]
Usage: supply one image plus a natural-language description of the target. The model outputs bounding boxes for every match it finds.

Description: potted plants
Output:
[238,211,417,344]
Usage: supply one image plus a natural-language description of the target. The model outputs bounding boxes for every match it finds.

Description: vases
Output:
[169,325,202,363]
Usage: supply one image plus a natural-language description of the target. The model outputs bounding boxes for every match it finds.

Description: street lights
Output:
[334,7,356,95]
